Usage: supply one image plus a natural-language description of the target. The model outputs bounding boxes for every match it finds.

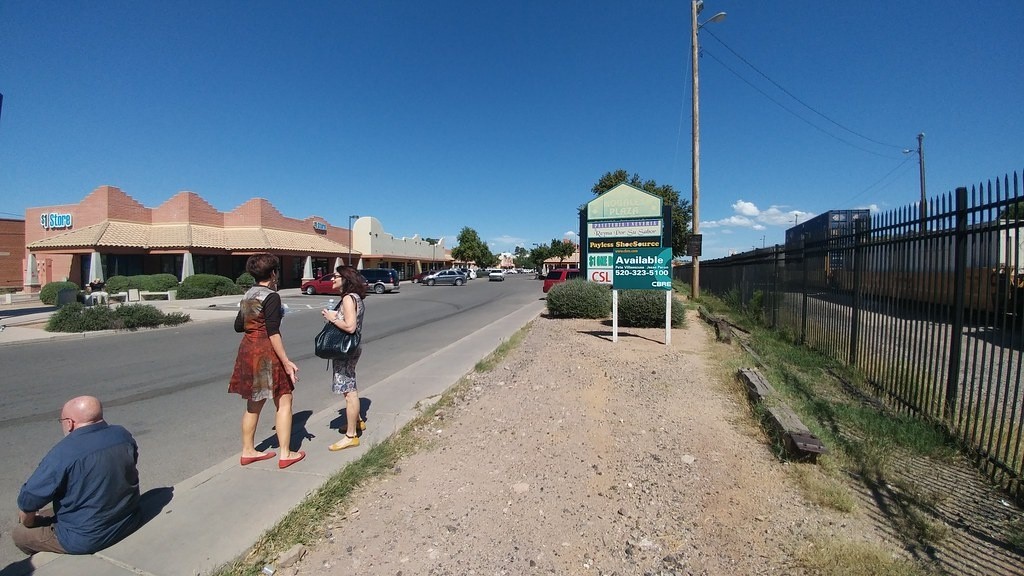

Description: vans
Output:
[356,269,400,294]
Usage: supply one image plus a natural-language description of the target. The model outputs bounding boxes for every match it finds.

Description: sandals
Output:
[340,420,366,432]
[328,433,360,451]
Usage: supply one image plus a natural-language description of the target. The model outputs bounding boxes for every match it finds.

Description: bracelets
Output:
[332,318,338,324]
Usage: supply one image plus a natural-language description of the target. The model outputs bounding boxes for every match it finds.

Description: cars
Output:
[411,265,506,286]
[301,273,342,296]
[543,267,581,293]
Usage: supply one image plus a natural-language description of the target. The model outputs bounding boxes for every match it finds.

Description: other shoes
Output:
[278,450,306,468]
[240,451,277,465]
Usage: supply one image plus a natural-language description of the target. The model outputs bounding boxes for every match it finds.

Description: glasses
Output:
[57,418,75,423]
[331,274,342,279]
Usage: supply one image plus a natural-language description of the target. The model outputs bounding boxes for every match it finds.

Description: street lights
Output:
[349,215,359,268]
[691,0,727,303]
[904,132,928,235]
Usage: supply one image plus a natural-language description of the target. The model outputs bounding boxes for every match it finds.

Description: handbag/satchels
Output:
[314,294,362,360]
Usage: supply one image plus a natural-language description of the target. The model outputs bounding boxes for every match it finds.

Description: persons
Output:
[12,396,138,555]
[226,254,306,468]
[322,266,369,451]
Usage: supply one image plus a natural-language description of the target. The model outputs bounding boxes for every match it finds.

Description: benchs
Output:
[140,290,177,302]
[85,292,128,305]
